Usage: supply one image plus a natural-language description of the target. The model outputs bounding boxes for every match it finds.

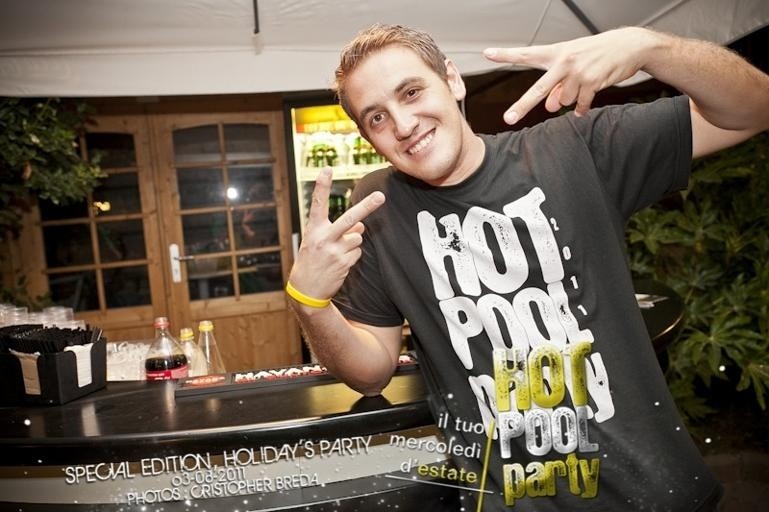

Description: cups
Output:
[0,302,87,331]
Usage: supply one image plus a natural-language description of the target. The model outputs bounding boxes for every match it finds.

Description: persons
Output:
[240,182,277,247]
[281,23,769,511]
[68,226,123,310]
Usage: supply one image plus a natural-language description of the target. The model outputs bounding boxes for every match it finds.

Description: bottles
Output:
[303,130,388,223]
[145,317,228,382]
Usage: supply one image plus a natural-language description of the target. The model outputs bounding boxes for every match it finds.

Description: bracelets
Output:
[284,277,333,309]
